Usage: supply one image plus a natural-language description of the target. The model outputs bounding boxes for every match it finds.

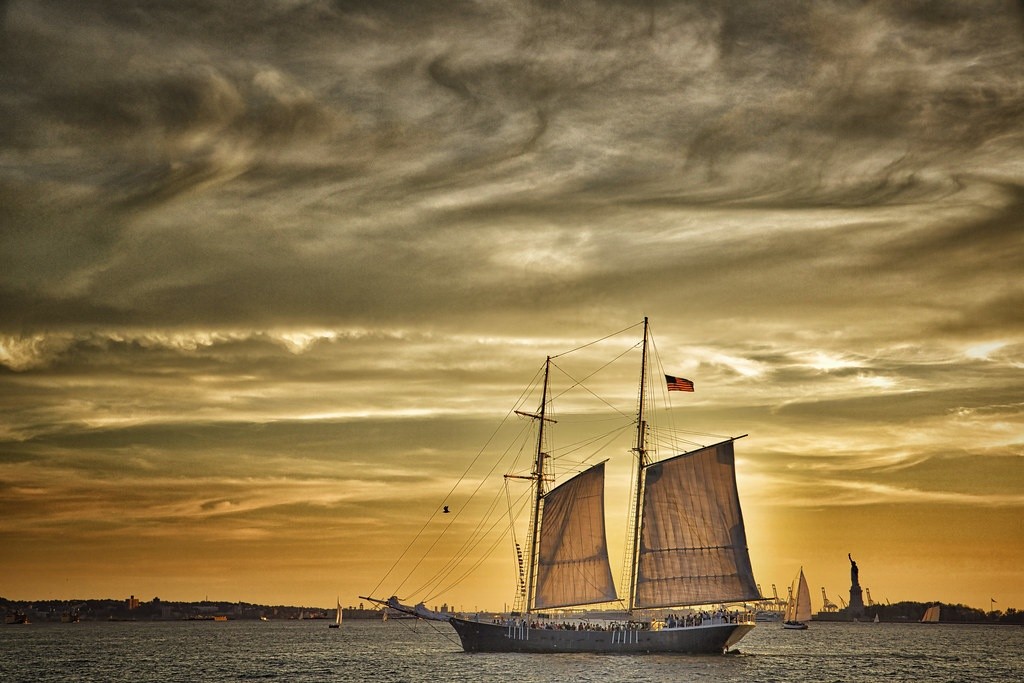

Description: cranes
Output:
[865,588,874,606]
[754,583,838,612]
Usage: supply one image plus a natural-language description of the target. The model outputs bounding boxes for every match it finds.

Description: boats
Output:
[752,609,777,622]
[258,616,270,622]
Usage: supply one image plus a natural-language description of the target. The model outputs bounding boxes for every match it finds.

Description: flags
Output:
[665,374,695,392]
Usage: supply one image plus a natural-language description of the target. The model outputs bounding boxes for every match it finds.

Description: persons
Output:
[493,617,635,632]
[848,552,861,592]
[667,609,741,628]
[651,618,656,630]
[474,613,479,622]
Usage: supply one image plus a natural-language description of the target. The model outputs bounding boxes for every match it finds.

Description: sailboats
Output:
[781,565,812,629]
[381,608,389,624]
[873,612,880,624]
[358,313,779,656]
[917,600,941,623]
[328,595,343,628]
